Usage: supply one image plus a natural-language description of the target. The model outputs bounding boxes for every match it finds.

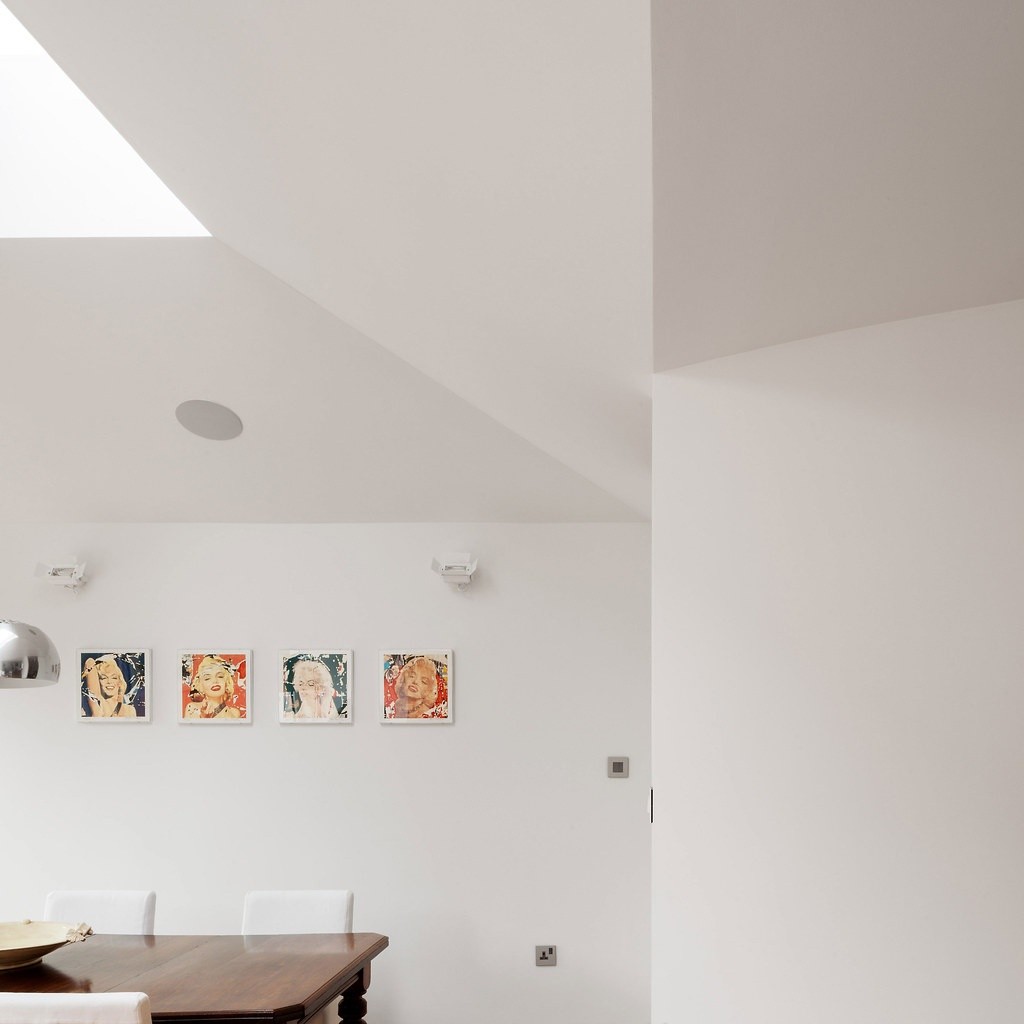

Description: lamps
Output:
[43,562,86,587]
[0,618,61,688]
[430,552,480,587]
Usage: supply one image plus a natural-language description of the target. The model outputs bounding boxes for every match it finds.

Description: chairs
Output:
[241,889,354,932]
[0,992,152,1024]
[42,890,157,936]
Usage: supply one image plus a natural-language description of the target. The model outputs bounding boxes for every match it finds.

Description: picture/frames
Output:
[76,648,153,723]
[178,648,252,724]
[377,650,456,724]
[277,647,354,724]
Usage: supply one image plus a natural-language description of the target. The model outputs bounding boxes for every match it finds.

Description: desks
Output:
[0,932,390,1024]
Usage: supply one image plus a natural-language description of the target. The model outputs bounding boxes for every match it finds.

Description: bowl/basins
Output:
[0,920,94,969]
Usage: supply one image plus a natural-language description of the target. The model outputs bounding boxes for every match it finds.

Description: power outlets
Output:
[536,945,558,967]
